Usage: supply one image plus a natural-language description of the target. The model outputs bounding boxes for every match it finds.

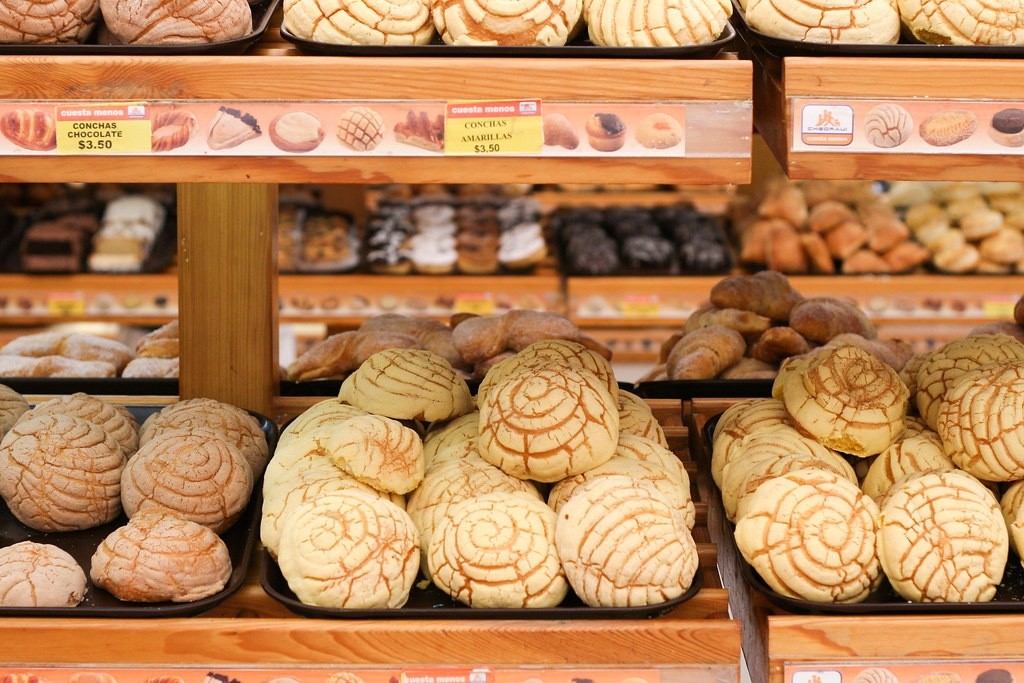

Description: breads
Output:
[152,105,445,151]
[274,184,727,276]
[279,309,613,382]
[260,338,697,612]
[543,112,683,152]
[862,103,1024,149]
[0,108,58,152]
[741,181,1024,277]
[0,0,1024,46]
[0,384,270,609]
[19,192,172,275]
[708,332,1023,604]
[0,320,180,380]
[635,268,1024,380]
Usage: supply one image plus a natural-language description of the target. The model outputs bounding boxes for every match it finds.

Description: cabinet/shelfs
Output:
[0,36,1024,683]
